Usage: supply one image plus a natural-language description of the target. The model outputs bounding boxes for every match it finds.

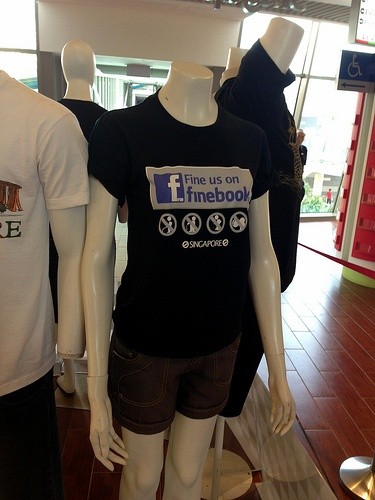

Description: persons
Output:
[0,18,305,500]
[297,129,307,175]
[326,189,332,205]
